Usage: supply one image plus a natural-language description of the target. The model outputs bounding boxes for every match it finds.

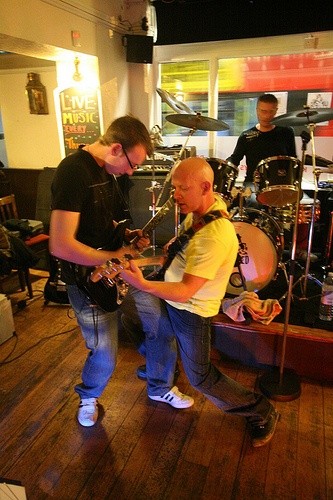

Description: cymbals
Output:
[156,87,197,116]
[270,107,333,126]
[166,114,230,132]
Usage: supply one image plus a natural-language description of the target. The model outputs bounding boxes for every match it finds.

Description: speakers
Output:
[126,34,153,64]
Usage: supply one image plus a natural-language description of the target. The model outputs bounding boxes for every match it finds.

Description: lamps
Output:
[25,73,48,115]
[72,56,82,81]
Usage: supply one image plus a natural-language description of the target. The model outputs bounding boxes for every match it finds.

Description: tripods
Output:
[261,124,333,302]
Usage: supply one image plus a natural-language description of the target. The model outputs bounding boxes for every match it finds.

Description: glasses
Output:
[121,146,141,171]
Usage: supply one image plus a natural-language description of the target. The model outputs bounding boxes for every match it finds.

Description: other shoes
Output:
[249,406,281,448]
[137,363,180,379]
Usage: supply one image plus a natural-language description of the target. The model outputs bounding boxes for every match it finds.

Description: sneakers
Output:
[77,397,99,428]
[149,386,194,409]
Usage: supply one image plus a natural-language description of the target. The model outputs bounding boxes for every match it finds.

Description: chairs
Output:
[0,194,49,299]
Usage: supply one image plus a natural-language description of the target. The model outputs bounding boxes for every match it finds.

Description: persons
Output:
[225,93,317,263]
[121,158,280,448]
[49,115,194,427]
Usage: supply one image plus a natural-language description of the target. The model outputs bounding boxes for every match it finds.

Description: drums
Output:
[265,198,320,223]
[205,158,239,207]
[254,155,304,208]
[225,208,283,298]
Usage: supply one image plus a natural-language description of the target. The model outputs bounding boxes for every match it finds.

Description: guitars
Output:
[88,236,187,283]
[79,188,177,313]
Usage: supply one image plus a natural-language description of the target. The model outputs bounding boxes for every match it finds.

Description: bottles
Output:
[319,272,333,322]
